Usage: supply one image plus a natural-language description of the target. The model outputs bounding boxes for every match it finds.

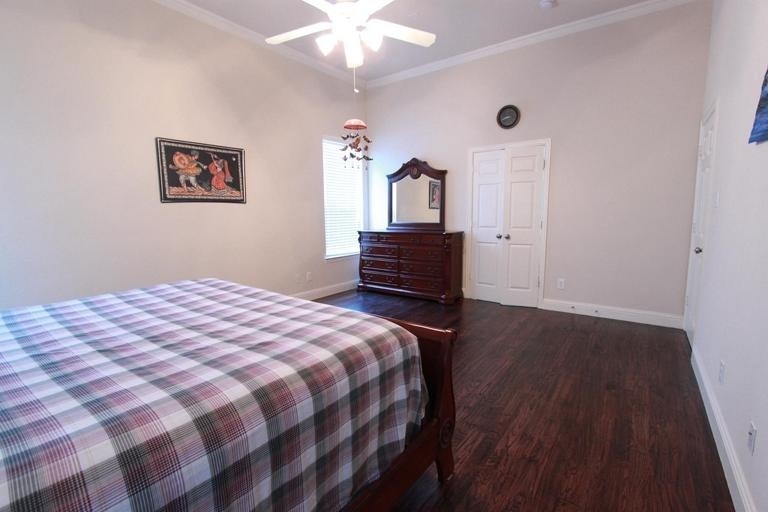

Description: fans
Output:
[260,0,438,50]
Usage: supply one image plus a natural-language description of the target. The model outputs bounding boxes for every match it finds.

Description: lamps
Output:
[314,23,382,68]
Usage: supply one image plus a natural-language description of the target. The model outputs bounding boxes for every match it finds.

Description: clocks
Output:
[496,104,520,128]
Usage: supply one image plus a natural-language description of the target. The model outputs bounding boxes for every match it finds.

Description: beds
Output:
[1,273,459,510]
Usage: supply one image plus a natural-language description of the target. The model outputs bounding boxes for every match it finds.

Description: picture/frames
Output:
[427,180,442,210]
[152,136,248,205]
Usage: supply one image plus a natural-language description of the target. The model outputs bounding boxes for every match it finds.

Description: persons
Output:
[206,151,234,194]
[169,150,208,193]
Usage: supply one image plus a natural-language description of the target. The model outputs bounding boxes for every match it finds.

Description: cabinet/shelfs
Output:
[356,227,464,307]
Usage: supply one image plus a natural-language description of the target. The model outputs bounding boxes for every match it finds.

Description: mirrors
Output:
[387,157,447,229]
